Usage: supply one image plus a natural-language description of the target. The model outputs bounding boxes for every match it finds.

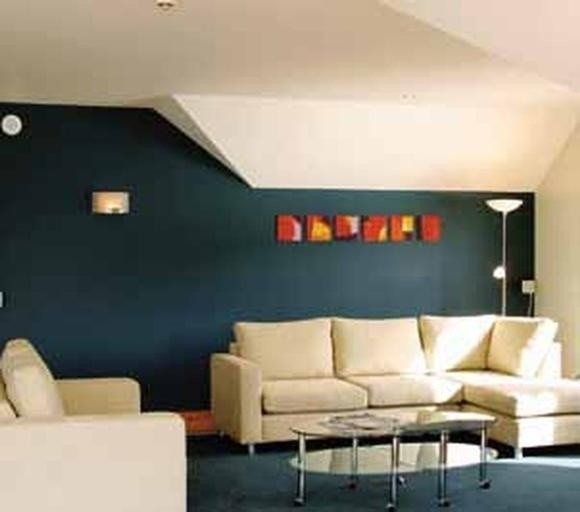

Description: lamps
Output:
[90,191,130,215]
[485,199,524,316]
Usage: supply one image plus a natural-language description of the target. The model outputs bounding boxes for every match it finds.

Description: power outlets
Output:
[522,280,536,294]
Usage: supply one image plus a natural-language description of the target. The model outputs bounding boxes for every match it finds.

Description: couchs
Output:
[209,351,465,457]
[429,367,580,458]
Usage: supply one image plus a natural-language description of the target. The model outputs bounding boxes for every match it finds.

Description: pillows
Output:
[2,338,64,417]
[419,314,494,372]
[331,315,429,377]
[232,318,332,378]
[485,318,556,375]
[0,378,188,512]
